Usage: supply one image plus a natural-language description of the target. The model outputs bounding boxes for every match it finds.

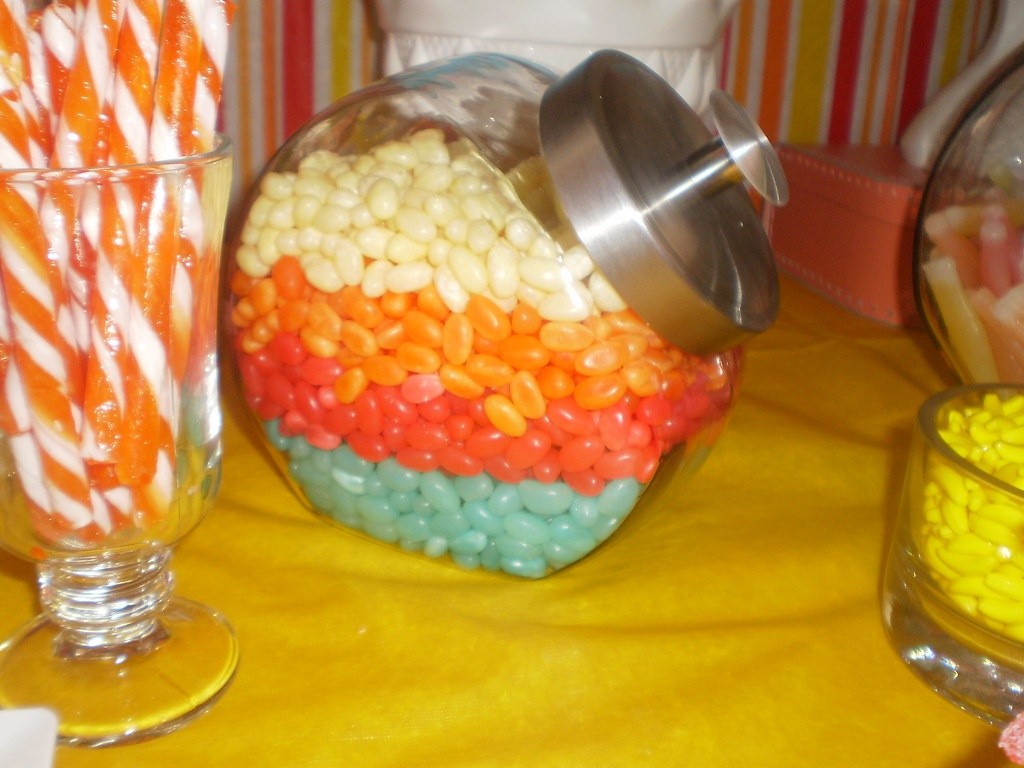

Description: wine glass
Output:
[0,128,241,750]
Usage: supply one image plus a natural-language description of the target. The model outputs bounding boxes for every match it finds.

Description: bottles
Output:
[224,50,792,585]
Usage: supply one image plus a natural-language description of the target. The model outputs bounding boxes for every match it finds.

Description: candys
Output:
[232,122,743,576]
[0,0,240,552]
[916,161,1024,653]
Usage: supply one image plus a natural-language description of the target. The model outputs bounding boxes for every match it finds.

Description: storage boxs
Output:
[774,140,987,328]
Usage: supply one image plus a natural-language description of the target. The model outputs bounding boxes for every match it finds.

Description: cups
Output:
[878,384,1024,729]
